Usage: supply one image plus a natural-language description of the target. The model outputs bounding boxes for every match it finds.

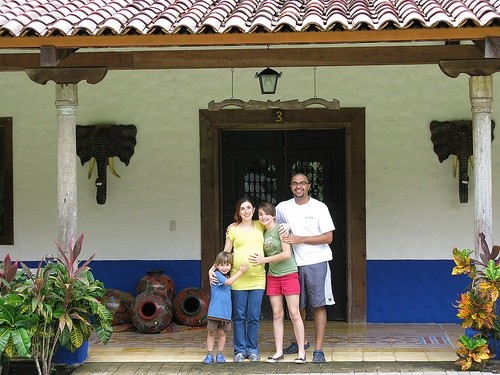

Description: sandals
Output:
[203,355,214,364]
[216,354,225,363]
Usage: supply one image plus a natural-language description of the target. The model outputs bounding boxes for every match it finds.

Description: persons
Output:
[209,198,291,364]
[274,174,336,363]
[247,203,307,363]
[203,252,249,363]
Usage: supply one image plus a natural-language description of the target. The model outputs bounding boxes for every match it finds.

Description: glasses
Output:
[292,182,310,186]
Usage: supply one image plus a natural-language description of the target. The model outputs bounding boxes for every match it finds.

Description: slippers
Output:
[313,350,325,361]
[283,342,310,354]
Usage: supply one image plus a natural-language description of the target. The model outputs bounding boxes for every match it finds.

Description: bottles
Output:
[171,287,210,327]
[96,288,137,326]
[137,271,175,303]
[128,290,174,334]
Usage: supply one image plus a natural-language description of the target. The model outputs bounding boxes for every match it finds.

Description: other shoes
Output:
[247,353,260,362]
[234,353,245,363]
[266,354,284,363]
[295,354,306,363]
